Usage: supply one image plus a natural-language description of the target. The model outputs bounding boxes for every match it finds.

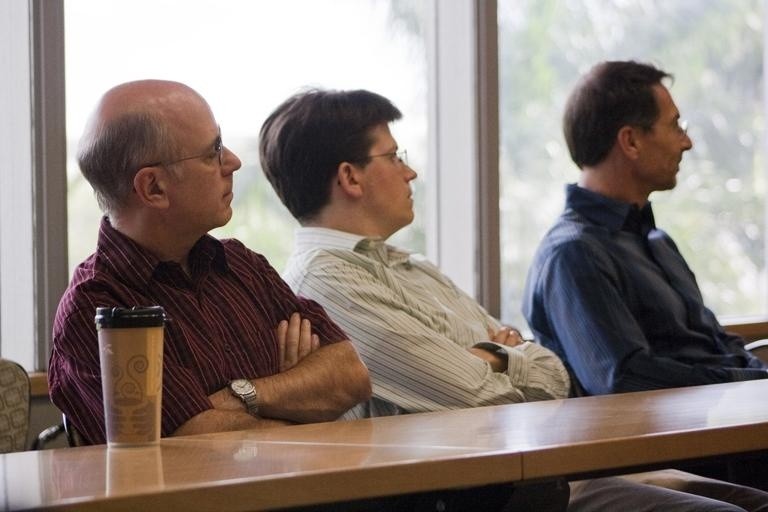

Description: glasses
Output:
[368,150,408,169]
[145,125,222,167]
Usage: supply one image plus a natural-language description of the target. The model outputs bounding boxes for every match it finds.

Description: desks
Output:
[0,378,768,512]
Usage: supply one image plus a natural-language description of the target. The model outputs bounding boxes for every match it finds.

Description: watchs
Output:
[227,377,259,415]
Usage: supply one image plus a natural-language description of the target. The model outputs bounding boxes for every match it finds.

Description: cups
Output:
[94,305,167,448]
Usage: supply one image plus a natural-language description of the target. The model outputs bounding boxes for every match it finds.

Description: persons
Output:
[518,57,768,486]
[256,83,766,510]
[46,76,571,511]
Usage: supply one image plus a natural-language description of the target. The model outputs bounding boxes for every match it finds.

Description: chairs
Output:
[0,358,32,455]
